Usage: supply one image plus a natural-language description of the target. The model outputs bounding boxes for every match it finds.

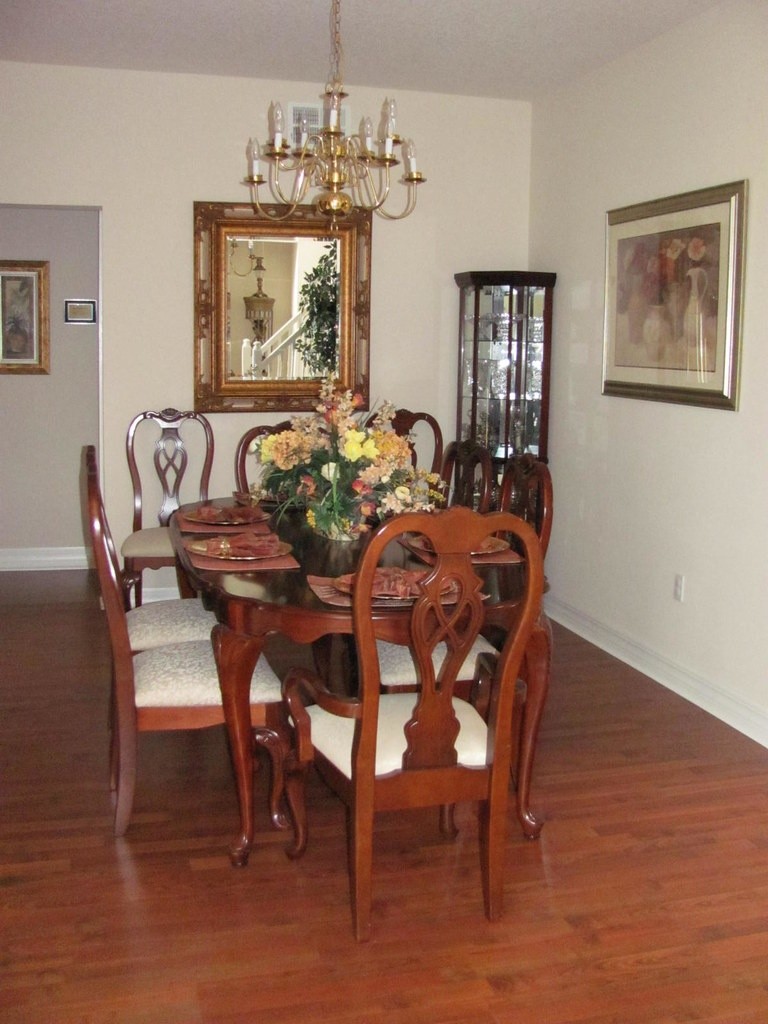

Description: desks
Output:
[170,494,552,869]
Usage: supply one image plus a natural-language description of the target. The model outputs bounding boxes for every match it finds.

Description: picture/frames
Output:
[0,258,52,375]
[603,177,748,412]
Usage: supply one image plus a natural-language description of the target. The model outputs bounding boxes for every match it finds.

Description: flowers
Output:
[261,367,448,534]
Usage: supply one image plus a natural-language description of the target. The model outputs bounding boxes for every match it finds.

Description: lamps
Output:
[226,236,254,276]
[252,256,268,297]
[236,0,426,239]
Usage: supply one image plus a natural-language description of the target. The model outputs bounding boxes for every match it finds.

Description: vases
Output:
[301,526,361,577]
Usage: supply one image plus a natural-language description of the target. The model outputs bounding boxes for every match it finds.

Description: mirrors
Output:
[188,201,371,420]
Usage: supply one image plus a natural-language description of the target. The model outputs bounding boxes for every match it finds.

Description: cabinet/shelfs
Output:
[453,272,558,479]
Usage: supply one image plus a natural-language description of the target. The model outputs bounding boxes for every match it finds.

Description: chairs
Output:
[119,409,214,607]
[236,409,554,558]
[281,504,546,944]
[85,445,299,835]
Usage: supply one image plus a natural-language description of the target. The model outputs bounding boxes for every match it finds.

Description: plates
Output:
[334,568,455,600]
[183,510,271,524]
[407,535,510,554]
[185,539,293,560]
[260,497,301,502]
